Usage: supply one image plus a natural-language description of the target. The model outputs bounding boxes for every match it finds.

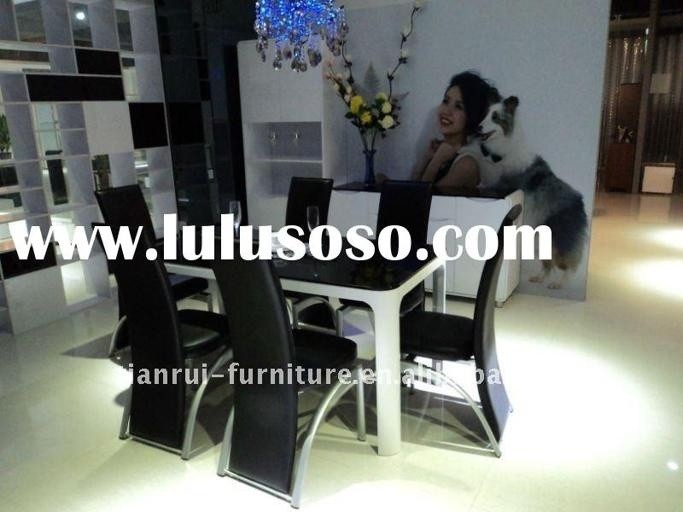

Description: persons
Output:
[411,68,502,190]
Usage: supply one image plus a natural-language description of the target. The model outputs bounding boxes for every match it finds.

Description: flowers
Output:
[323,3,422,151]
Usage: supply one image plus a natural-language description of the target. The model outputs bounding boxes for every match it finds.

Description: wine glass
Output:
[305,246,326,278]
[306,204,319,242]
[260,124,304,160]
[226,200,242,239]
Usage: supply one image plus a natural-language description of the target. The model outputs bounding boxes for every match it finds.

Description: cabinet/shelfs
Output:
[237,37,346,229]
[0,0,176,335]
[153,0,238,225]
[605,142,635,193]
[641,162,675,195]
[616,83,642,143]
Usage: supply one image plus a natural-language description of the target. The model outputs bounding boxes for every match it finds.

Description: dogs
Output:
[458,87,591,291]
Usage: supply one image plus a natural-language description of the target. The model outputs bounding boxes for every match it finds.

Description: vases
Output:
[362,149,379,187]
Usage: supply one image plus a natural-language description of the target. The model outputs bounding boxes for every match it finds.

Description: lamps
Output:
[254,1,346,73]
